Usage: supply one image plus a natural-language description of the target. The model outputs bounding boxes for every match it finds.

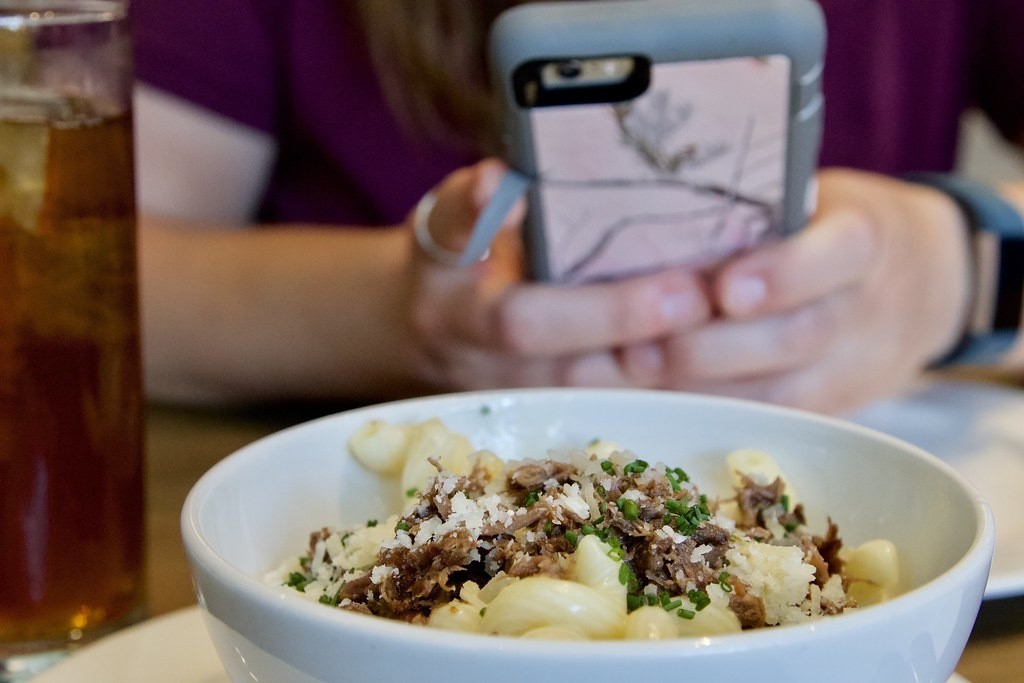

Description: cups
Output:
[1,0,147,658]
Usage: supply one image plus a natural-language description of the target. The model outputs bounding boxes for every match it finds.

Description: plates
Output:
[22,605,231,683]
[841,384,1024,600]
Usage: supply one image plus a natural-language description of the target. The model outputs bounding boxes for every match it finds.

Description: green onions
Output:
[286,458,800,620]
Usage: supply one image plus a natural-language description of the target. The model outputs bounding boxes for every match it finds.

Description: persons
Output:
[29,0,1024,419]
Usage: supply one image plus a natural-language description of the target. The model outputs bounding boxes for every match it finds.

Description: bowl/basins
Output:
[177,388,991,683]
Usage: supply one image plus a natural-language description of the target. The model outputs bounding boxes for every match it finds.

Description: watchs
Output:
[926,176,1024,371]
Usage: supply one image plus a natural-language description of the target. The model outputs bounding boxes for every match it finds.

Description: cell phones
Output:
[486,0,829,285]
